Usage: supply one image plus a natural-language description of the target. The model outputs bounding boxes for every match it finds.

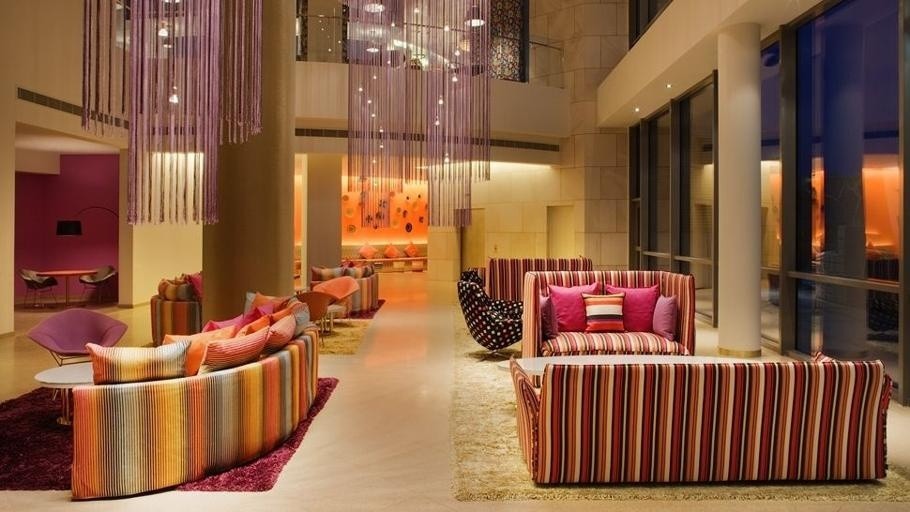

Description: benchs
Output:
[296,244,427,272]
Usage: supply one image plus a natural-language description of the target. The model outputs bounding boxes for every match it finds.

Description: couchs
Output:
[71,321,321,499]
[521,269,697,359]
[147,295,205,347]
[508,350,900,487]
[482,255,594,302]
[310,272,379,317]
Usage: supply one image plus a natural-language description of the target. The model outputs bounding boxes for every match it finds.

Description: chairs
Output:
[462,270,482,286]
[292,290,338,337]
[458,283,524,361]
[312,273,360,339]
[18,267,59,309]
[27,310,126,366]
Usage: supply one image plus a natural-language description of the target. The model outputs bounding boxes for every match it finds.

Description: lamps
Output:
[464,0,485,27]
[81,0,264,227]
[366,32,379,53]
[364,0,386,13]
[348,0,492,229]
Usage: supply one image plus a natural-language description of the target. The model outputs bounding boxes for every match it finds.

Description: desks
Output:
[496,354,766,388]
[38,270,97,305]
[34,362,95,426]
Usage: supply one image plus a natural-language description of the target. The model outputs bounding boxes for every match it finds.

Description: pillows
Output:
[358,241,419,259]
[651,293,680,342]
[539,294,558,340]
[583,292,627,336]
[86,289,312,389]
[155,269,202,300]
[605,282,660,333]
[547,281,600,332]
[310,262,379,283]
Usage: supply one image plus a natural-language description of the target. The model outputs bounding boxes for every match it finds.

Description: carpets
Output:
[0,377,341,493]
[450,288,910,503]
